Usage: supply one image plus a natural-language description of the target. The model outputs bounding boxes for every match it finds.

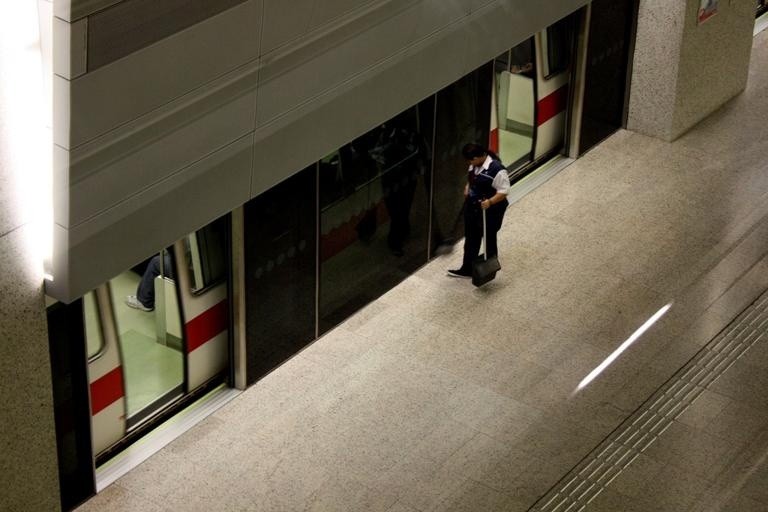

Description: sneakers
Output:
[447,268,472,280]
[125,295,154,312]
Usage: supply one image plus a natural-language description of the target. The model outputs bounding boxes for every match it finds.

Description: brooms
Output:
[433,193,470,255]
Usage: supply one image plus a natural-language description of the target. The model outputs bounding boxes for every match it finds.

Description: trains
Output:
[81,0,766,468]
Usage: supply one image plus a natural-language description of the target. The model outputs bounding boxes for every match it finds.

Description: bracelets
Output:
[488,199,492,208]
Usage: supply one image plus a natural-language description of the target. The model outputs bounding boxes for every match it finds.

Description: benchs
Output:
[154,266,192,352]
[498,65,539,141]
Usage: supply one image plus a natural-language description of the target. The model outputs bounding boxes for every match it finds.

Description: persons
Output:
[121,253,173,313]
[493,43,533,77]
[442,143,511,279]
[380,129,419,258]
